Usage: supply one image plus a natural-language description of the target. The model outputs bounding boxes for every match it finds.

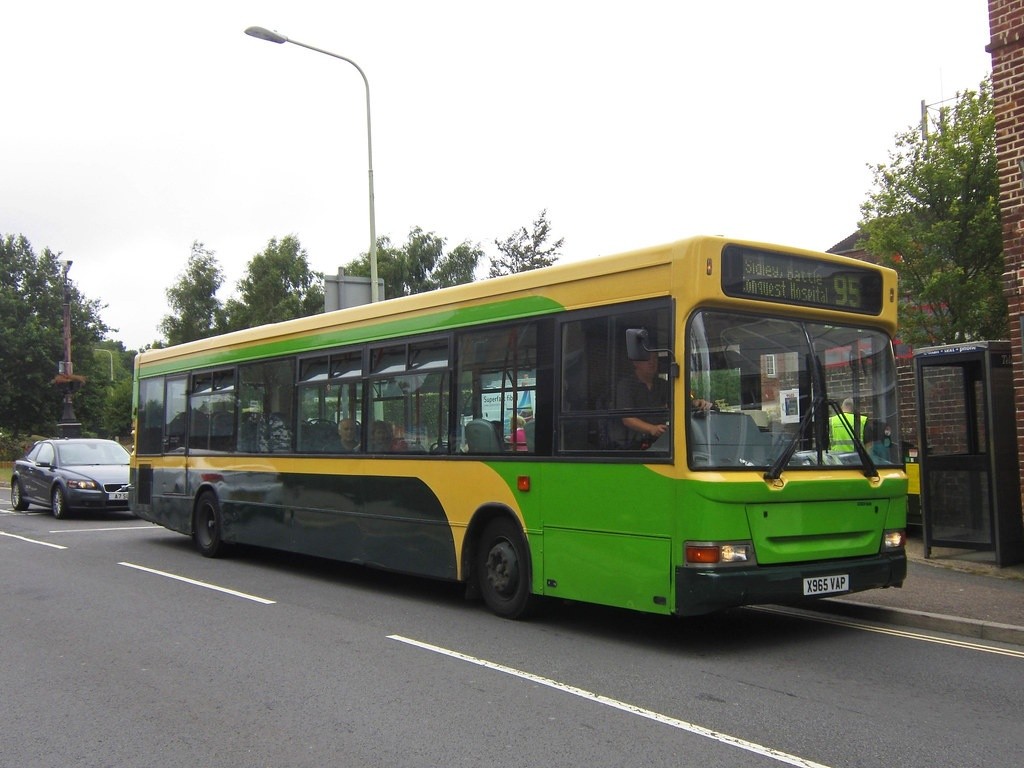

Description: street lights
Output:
[244,27,378,307]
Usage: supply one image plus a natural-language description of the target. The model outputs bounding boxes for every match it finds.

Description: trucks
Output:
[461,378,536,437]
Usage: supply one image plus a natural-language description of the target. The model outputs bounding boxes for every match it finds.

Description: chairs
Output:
[169,411,449,454]
[464,419,535,453]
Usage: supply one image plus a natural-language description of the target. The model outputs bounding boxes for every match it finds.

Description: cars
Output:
[10,438,133,520]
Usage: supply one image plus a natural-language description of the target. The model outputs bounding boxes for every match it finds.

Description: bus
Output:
[129,234,910,623]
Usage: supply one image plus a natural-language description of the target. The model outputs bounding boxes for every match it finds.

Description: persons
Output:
[822,395,879,458]
[328,418,363,452]
[617,334,712,457]
[369,419,397,451]
[505,415,527,447]
